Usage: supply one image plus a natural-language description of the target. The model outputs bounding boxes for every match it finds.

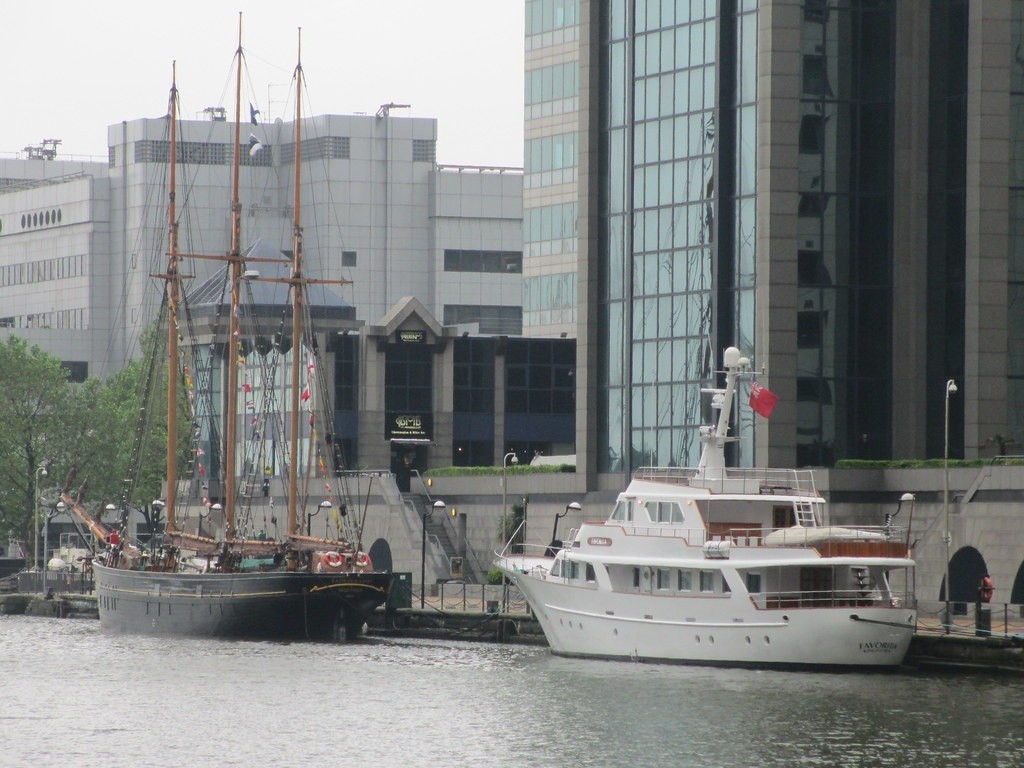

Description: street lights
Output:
[90,504,116,593]
[884,492,915,580]
[420,501,446,609]
[502,452,518,611]
[307,501,333,569]
[35,466,48,578]
[150,501,164,551]
[547,502,581,555]
[195,504,222,557]
[945,377,957,635]
[43,502,65,579]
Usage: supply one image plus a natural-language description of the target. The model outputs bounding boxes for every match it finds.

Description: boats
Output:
[495,344,923,671]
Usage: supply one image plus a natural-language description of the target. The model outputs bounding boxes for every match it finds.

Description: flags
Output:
[749,380,779,420]
[250,104,260,126]
[249,133,262,156]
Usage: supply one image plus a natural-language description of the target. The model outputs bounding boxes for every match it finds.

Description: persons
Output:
[980,572,993,603]
[258,530,266,540]
[273,548,283,564]
[110,544,122,568]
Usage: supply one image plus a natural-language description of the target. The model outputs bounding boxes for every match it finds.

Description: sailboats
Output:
[89,12,400,640]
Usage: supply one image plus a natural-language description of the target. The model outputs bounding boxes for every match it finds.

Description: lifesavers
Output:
[353,551,369,568]
[982,576,994,604]
[324,550,343,568]
